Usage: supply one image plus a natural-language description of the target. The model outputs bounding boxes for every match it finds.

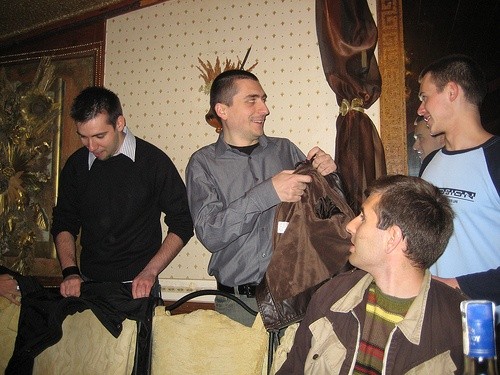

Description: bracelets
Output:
[62,266,80,280]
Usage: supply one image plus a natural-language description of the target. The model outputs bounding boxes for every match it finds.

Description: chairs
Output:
[0,293,22,375]
[32,279,142,375]
[151,289,274,375]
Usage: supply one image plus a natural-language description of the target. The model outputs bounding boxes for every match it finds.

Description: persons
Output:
[0,273,21,306]
[185,69,337,328]
[50,86,194,299]
[275,174,475,375]
[413,116,446,163]
[412,53,500,328]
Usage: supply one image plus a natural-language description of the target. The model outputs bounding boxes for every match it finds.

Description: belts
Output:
[217,281,258,297]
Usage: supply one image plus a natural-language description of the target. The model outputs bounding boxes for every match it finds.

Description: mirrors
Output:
[400,0,500,179]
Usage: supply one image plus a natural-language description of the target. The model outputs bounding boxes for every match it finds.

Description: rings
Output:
[17,285,19,290]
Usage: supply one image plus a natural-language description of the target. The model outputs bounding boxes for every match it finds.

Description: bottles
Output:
[460,300,498,375]
